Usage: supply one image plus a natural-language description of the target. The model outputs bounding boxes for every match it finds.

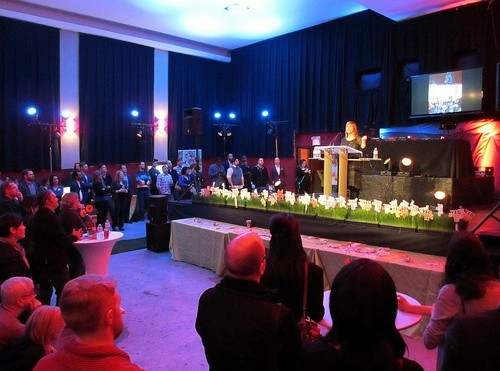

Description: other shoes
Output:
[112,226,118,231]
[119,226,124,231]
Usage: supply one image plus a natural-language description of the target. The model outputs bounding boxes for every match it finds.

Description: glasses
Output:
[18,291,38,301]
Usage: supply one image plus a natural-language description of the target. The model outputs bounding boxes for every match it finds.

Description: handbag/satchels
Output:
[174,181,182,191]
[298,316,321,347]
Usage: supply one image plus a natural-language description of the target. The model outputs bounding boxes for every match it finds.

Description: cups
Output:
[104,229,109,239]
[246,219,252,227]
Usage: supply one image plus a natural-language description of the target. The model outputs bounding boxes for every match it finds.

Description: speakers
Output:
[146,195,171,253]
[182,107,202,135]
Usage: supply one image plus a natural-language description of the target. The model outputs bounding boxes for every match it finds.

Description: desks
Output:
[170,214,449,337]
[73,231,124,278]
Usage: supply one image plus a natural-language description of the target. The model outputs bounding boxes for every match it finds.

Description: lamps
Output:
[382,157,393,170]
[129,107,143,122]
[135,127,144,138]
[53,126,62,140]
[438,121,456,134]
[259,108,273,120]
[226,127,233,137]
[483,167,495,177]
[217,126,226,137]
[266,126,277,138]
[428,190,450,212]
[214,112,224,122]
[398,155,416,172]
[227,112,238,122]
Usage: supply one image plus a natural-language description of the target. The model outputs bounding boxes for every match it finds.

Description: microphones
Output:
[329,132,341,146]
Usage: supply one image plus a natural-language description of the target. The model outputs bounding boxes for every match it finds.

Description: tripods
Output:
[177,135,207,201]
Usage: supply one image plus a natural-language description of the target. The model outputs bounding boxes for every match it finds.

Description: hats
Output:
[150,159,159,162]
[94,171,102,176]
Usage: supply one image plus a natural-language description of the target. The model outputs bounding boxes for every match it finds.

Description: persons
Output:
[195,231,309,371]
[32,275,146,371]
[397,230,500,371]
[311,258,424,371]
[295,159,311,194]
[340,120,367,199]
[332,158,338,198]
[259,213,325,322]
[0,153,133,371]
[135,154,286,222]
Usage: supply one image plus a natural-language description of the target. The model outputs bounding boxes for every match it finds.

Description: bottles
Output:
[97,224,103,240]
[373,148,378,159]
[92,223,97,239]
[105,220,110,233]
